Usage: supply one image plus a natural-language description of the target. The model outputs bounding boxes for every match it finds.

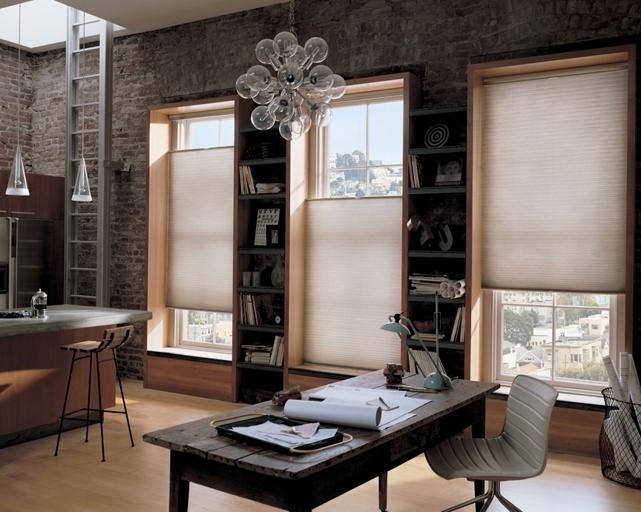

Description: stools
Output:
[54,324,135,462]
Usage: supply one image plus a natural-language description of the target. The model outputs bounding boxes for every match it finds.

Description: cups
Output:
[251,272,262,288]
[242,271,252,288]
[385,362,403,384]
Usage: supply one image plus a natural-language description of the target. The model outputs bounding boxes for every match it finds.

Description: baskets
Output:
[600,387,641,490]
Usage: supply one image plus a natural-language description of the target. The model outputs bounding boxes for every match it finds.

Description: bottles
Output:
[32,288,49,320]
[269,254,285,289]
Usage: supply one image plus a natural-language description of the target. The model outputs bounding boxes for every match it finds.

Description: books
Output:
[409,332,446,343]
[435,157,463,186]
[241,335,285,368]
[409,154,424,189]
[409,271,449,296]
[240,292,262,327]
[254,207,281,246]
[408,346,448,379]
[239,165,285,195]
[450,305,467,343]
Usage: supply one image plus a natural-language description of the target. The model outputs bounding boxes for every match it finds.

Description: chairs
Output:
[425,374,559,512]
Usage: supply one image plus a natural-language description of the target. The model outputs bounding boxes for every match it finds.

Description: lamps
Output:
[381,314,451,390]
[71,11,93,202]
[232,0,346,141]
[6,3,30,197]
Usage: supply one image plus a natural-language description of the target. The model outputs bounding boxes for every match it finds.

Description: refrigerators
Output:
[1,217,54,313]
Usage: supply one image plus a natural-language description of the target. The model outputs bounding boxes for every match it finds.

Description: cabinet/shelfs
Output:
[233,96,306,404]
[402,75,482,382]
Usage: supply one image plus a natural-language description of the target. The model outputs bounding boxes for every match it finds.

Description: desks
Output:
[143,365,501,512]
